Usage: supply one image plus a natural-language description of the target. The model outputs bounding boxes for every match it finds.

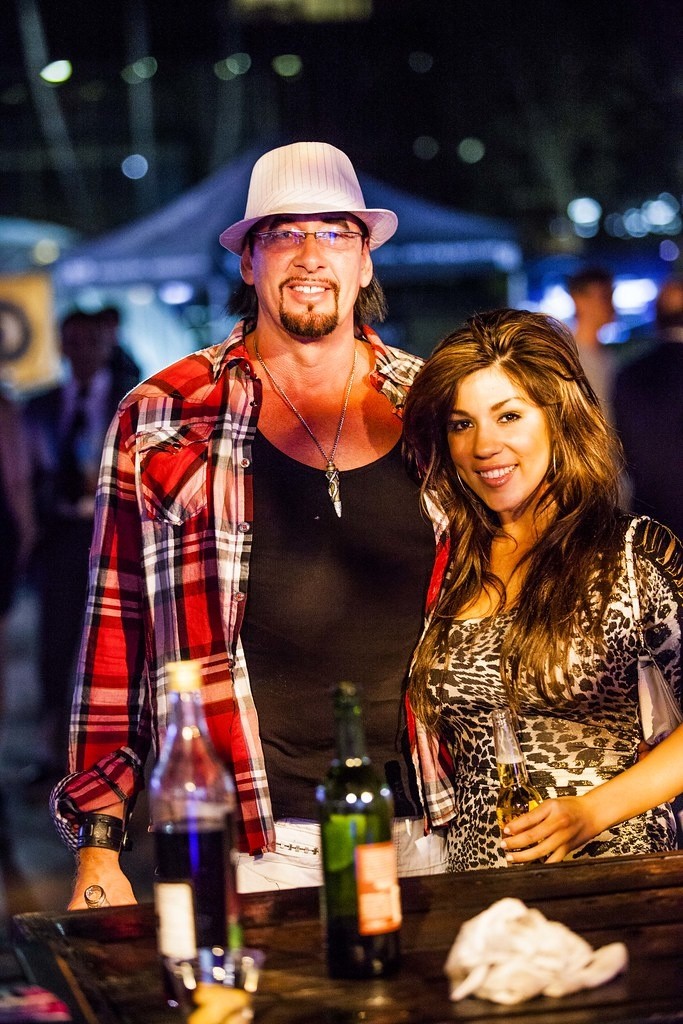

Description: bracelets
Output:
[78,812,128,852]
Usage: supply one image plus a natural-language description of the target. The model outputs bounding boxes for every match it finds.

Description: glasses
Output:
[251,229,369,251]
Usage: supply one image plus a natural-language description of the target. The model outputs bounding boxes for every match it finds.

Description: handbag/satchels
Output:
[624,514,683,746]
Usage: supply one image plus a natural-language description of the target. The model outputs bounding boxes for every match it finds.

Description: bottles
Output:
[321,679,406,980]
[84,885,111,908]
[490,709,547,866]
[147,660,241,1012]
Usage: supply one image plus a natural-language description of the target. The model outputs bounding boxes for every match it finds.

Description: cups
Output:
[164,947,267,1024]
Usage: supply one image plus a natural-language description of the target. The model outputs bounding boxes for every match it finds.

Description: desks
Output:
[0,848,682,1024]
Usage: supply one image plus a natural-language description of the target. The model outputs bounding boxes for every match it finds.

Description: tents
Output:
[0,147,519,400]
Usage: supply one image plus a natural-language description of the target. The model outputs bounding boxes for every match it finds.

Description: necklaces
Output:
[251,338,358,518]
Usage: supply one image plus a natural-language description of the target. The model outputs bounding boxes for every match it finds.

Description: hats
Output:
[220,141,398,252]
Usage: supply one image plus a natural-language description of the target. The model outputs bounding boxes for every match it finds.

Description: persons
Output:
[570,268,616,426]
[410,311,683,870]
[614,284,683,542]
[49,142,455,912]
[0,301,141,782]
